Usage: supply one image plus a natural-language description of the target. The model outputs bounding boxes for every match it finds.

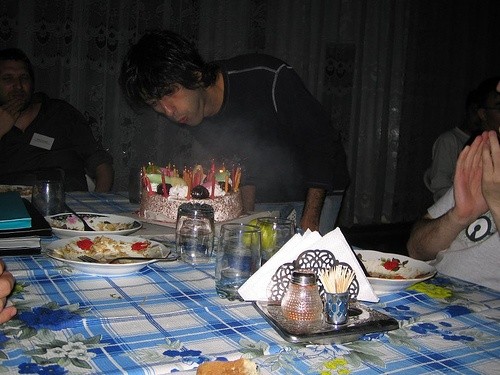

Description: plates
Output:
[44,233,170,275]
[353,249,438,293]
[0,184,35,197]
[44,211,142,239]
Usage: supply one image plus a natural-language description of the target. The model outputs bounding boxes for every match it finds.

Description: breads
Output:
[196,358,258,375]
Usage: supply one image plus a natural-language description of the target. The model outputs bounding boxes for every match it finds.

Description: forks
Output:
[77,255,177,264]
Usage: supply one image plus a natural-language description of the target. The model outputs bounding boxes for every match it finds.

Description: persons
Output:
[422,81,498,204]
[119,30,352,236]
[407,78,500,292]
[0,259,17,324]
[0,47,115,193]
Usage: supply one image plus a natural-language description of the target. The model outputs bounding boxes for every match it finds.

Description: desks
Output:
[0,192,500,375]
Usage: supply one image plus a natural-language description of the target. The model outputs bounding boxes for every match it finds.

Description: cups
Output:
[175,203,216,262]
[31,181,65,216]
[215,222,262,303]
[255,217,295,262]
[325,292,350,326]
[128,166,144,203]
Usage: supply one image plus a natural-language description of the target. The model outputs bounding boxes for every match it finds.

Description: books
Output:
[0,191,52,256]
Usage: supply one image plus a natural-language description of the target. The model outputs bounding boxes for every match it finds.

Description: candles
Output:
[138,156,242,200]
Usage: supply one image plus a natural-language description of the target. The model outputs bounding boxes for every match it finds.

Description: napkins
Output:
[238,225,380,302]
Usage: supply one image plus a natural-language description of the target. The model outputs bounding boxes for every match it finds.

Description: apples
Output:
[243,217,279,250]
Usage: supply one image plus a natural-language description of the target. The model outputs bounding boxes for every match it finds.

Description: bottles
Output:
[281,269,323,320]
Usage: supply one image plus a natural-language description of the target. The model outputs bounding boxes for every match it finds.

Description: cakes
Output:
[139,162,242,224]
